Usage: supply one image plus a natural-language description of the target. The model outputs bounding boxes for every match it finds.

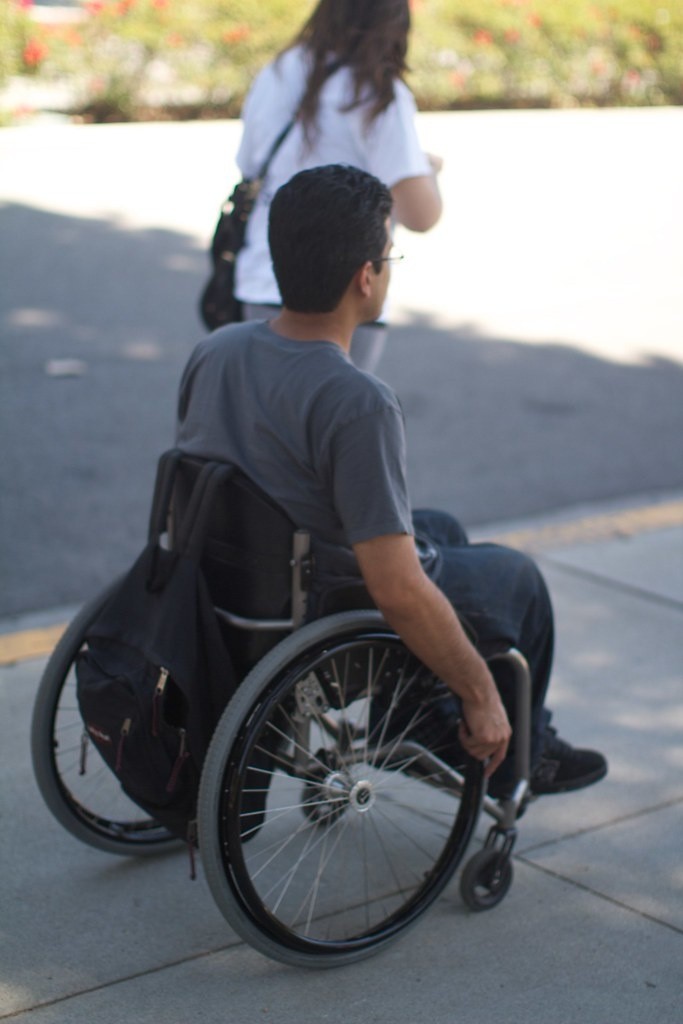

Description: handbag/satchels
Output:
[201,178,262,329]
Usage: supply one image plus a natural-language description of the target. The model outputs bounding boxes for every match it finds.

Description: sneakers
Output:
[486,742,607,798]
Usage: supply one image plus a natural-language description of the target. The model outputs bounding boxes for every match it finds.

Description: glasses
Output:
[374,246,404,267]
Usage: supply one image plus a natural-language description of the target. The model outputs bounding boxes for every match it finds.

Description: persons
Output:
[177,165,609,798]
[236,0,441,376]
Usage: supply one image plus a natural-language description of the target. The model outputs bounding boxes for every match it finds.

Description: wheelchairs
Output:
[30,445,542,971]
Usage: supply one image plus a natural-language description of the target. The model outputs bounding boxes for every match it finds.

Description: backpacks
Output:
[75,449,292,852]
